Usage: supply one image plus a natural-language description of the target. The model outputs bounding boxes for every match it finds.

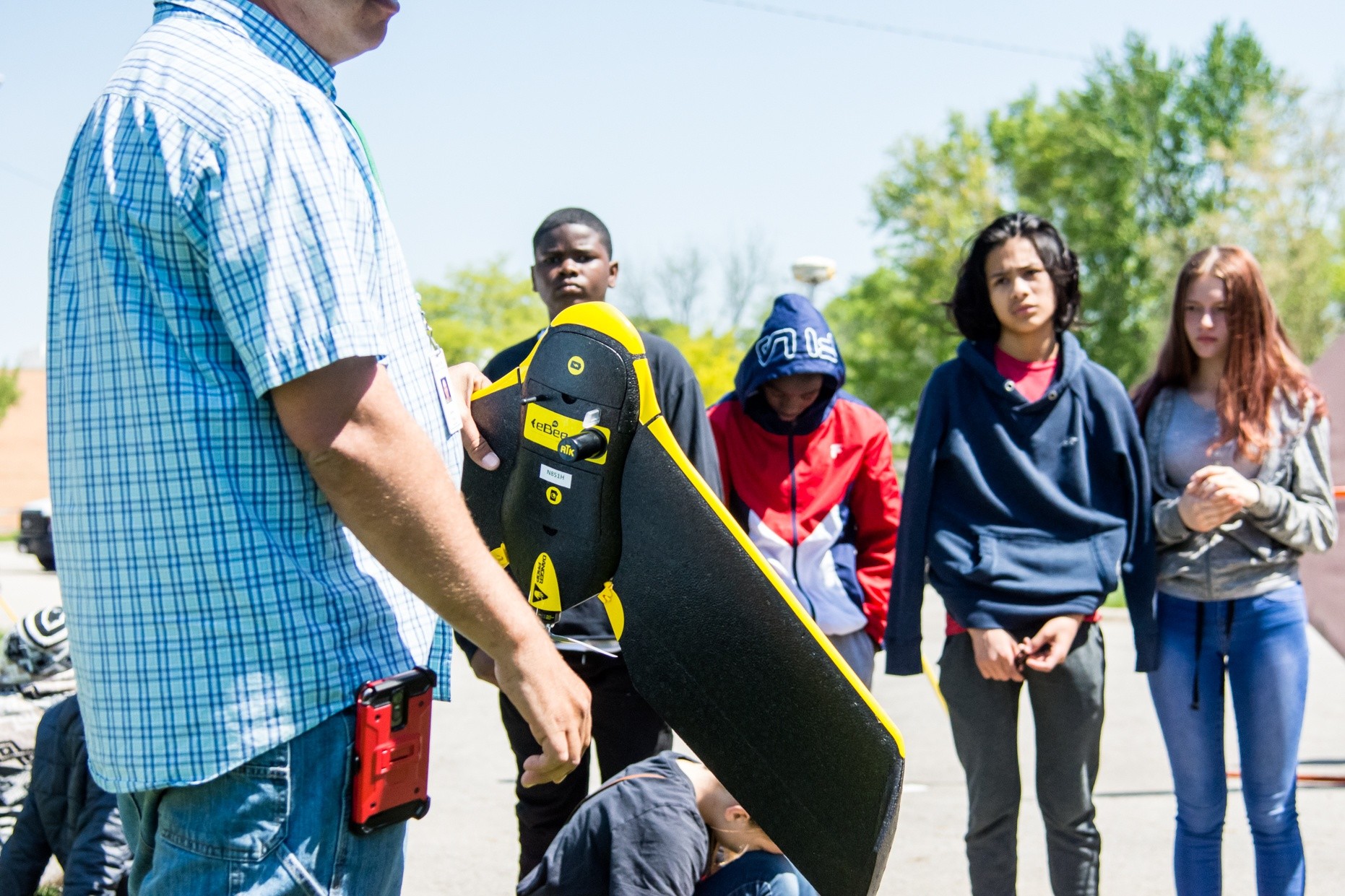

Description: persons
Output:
[1129,245,1336,896]
[453,211,723,883]
[706,292,901,693]
[884,210,1159,896]
[45,0,594,896]
[517,753,817,896]
[0,693,134,896]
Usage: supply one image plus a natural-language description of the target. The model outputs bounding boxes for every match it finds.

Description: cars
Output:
[16,503,57,571]
